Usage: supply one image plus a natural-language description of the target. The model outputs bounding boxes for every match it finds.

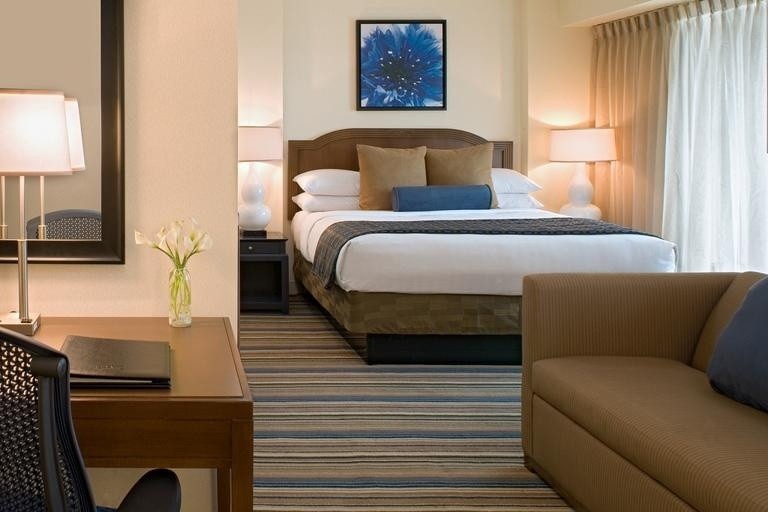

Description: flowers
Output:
[134,217,212,318]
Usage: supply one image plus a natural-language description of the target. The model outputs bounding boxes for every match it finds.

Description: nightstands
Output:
[239,231,290,316]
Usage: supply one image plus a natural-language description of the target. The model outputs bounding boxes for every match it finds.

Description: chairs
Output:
[0,329,182,512]
[29,209,103,240]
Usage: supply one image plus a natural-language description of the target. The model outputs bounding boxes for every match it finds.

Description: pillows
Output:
[294,169,362,196]
[428,143,498,211]
[292,192,360,211]
[496,195,545,209]
[492,167,542,193]
[358,145,425,208]
[708,278,768,411]
[394,185,492,211]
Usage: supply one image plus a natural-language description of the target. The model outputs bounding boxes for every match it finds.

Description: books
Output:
[58,335,172,390]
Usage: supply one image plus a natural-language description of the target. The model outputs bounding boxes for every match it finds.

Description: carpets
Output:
[239,293,574,512]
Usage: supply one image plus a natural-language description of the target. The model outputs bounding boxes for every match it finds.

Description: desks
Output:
[2,316,252,512]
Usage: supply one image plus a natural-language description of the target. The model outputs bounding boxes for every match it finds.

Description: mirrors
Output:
[0,0,124,264]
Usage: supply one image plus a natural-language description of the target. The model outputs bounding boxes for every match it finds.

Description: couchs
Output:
[521,272,768,512]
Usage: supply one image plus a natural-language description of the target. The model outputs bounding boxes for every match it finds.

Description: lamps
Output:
[238,126,284,238]
[38,98,86,239]
[550,128,617,220]
[1,88,73,336]
[1,176,10,240]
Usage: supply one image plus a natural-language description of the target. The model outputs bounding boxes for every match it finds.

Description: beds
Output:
[288,128,682,364]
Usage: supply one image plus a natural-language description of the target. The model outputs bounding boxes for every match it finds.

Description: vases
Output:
[167,268,193,327]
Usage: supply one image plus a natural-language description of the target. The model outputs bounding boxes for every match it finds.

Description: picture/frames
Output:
[356,20,447,111]
